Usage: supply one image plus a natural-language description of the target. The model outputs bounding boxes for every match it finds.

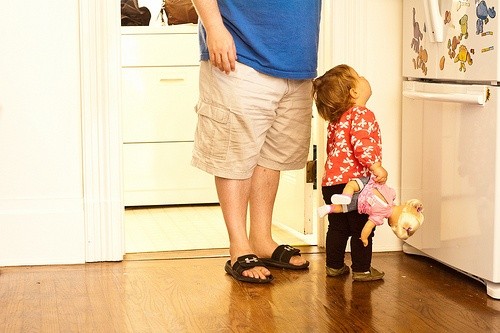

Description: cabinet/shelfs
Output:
[121,24,221,207]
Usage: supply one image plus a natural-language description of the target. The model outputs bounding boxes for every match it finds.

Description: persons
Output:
[319,174,425,247]
[311,65,390,281]
[191,0,323,283]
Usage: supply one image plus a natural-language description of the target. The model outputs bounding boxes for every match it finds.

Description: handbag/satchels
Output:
[160,0,198,25]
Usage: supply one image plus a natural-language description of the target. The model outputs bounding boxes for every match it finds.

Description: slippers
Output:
[224,254,274,283]
[259,244,310,269]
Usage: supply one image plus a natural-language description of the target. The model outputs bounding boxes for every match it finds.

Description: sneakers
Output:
[352,266,385,281]
[325,263,350,277]
